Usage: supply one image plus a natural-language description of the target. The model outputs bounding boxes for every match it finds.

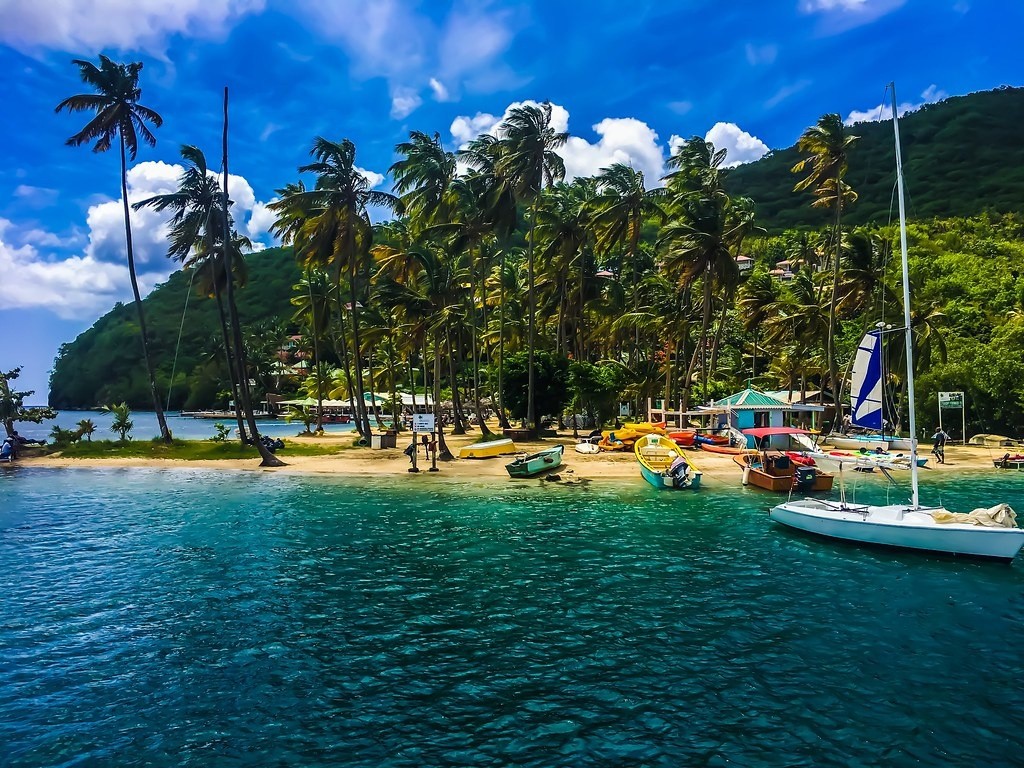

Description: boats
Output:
[459,438,516,459]
[994,459,1023,471]
[634,434,704,489]
[505,444,564,477]
[829,451,929,470]
[575,421,758,454]
[789,434,824,453]
[732,453,835,492]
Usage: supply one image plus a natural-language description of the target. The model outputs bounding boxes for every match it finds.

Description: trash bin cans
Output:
[370,427,397,449]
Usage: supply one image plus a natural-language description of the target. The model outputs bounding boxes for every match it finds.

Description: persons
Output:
[859,447,888,456]
[261,435,285,453]
[841,413,852,435]
[1,431,21,459]
[399,411,476,430]
[931,427,947,463]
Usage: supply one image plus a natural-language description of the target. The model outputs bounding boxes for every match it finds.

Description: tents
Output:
[276,392,433,415]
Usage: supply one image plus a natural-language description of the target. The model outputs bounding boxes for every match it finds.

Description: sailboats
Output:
[768,81,1024,566]
[826,329,918,451]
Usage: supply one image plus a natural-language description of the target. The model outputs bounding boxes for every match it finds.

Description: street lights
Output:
[875,321,891,441]
